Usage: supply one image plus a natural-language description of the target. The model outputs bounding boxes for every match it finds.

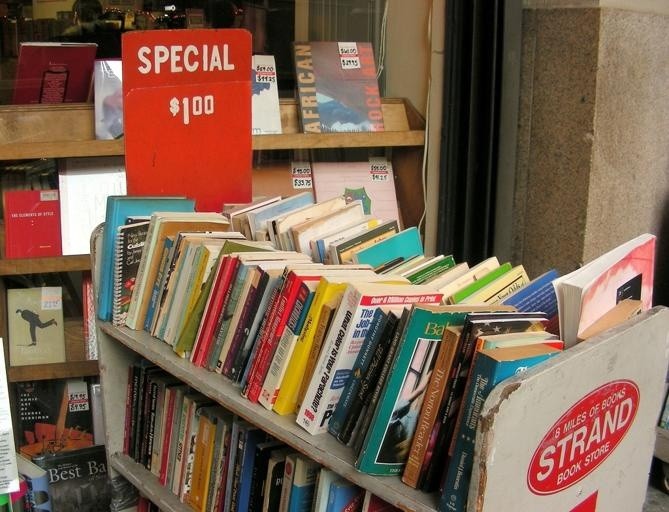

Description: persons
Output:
[55,0,122,57]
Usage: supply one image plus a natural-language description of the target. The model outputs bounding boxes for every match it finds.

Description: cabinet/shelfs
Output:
[1,97,432,511]
[90,221,668,511]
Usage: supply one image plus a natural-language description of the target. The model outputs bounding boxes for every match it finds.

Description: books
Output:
[2,39,668,512]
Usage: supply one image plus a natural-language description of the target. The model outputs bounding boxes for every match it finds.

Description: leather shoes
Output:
[29,343,36,346]
[52,319,57,326]
